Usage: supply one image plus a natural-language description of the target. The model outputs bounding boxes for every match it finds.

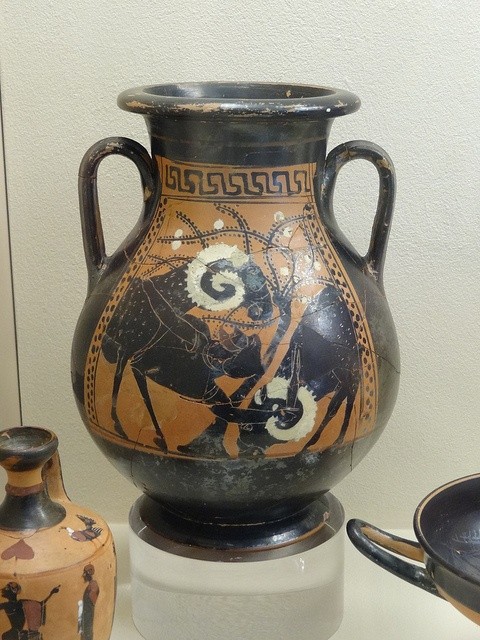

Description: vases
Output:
[0,421,119,640]
[345,471,480,627]
[70,79,401,563]
